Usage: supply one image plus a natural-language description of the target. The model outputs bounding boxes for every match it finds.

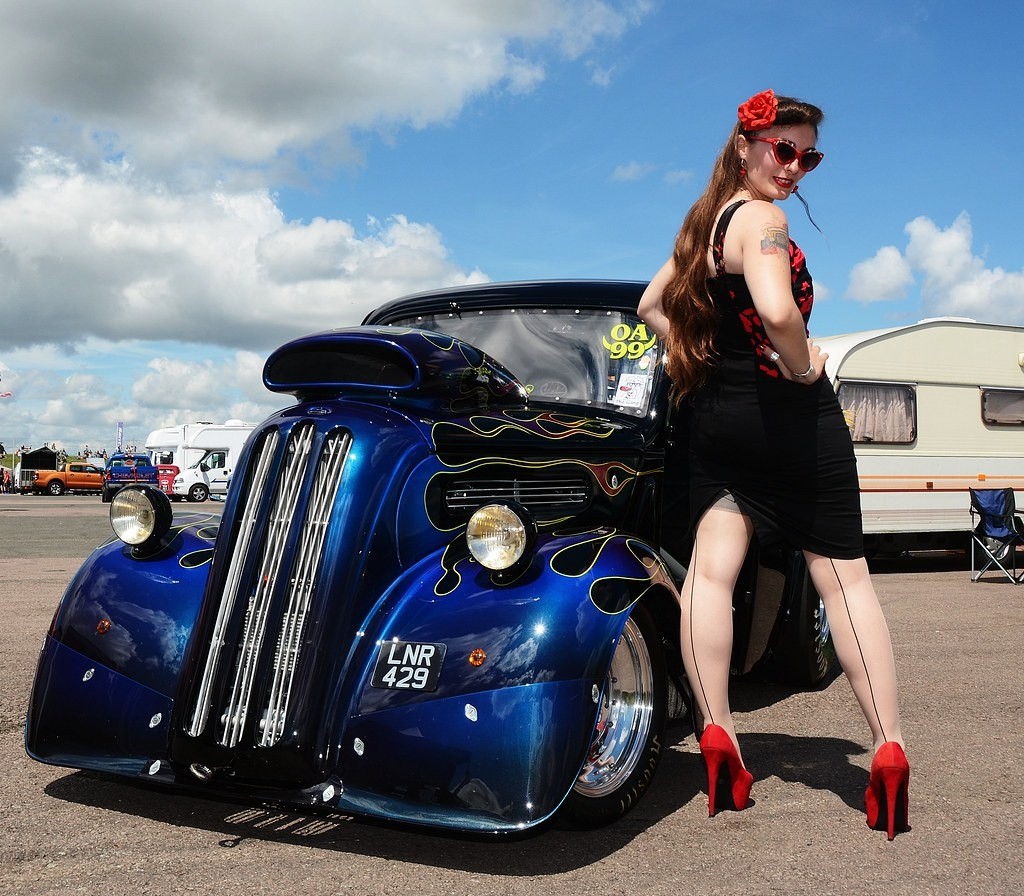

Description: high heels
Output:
[700,723,754,819]
[863,740,909,839]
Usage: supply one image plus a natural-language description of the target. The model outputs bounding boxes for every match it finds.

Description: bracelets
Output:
[793,362,813,379]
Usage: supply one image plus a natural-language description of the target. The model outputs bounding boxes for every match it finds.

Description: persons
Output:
[52,443,136,465]
[636,87,911,841]
[3,471,10,494]
[0,468,5,494]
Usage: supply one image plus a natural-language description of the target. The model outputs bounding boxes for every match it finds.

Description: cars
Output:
[27,280,844,839]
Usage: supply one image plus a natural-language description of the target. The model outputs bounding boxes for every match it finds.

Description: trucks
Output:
[145,417,259,501]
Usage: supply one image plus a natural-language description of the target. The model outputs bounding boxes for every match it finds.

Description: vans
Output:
[155,464,182,501]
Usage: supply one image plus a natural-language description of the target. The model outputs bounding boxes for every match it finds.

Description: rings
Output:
[770,352,780,362]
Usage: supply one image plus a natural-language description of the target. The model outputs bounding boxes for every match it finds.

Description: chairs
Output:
[968,487,1024,585]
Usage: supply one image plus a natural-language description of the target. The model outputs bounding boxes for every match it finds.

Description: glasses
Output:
[745,136,824,172]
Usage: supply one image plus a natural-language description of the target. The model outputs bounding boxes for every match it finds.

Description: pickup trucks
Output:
[32,462,108,495]
[102,452,159,503]
